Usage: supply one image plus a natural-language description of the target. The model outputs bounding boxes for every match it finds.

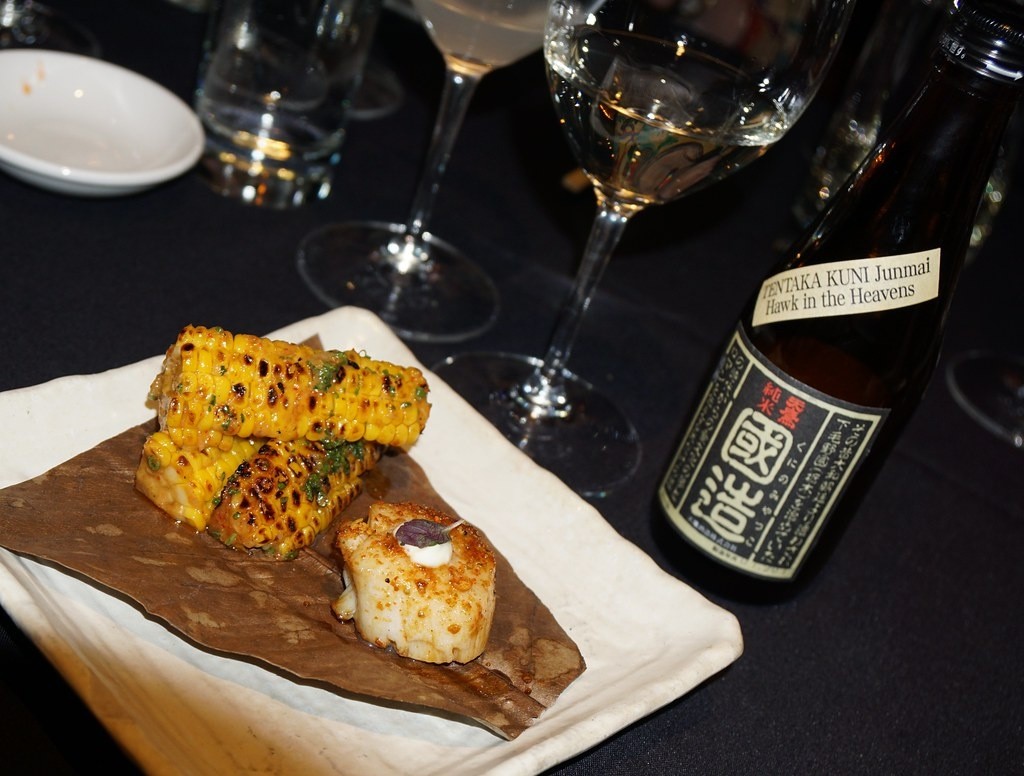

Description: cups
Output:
[194,0,371,209]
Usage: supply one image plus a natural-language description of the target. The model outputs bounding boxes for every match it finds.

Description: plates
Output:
[1,307,744,776]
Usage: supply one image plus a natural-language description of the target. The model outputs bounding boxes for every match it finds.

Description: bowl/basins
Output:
[0,49,205,196]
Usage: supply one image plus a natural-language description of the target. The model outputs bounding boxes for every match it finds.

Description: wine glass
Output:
[296,0,549,344]
[428,0,846,503]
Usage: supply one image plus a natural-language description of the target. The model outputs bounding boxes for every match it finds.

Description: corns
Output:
[140,324,433,563]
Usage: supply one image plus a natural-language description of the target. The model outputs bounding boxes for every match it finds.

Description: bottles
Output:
[648,2,1024,614]
